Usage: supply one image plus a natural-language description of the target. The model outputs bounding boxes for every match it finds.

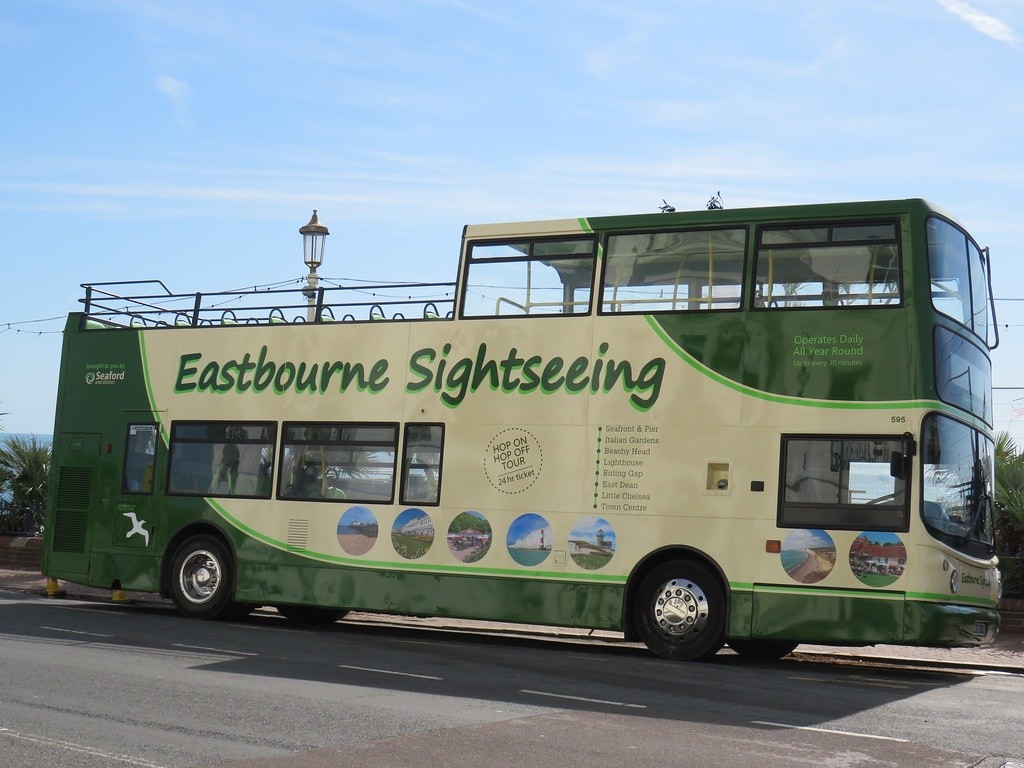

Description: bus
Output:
[41,198,1002,664]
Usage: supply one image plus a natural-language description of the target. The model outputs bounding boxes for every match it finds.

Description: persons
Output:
[453,537,484,551]
[854,560,905,578]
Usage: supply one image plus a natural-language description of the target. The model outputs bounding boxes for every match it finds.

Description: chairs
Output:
[325,468,347,499]
[84,304,455,329]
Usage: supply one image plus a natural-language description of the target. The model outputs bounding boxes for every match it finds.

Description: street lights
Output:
[299,209,330,324]
